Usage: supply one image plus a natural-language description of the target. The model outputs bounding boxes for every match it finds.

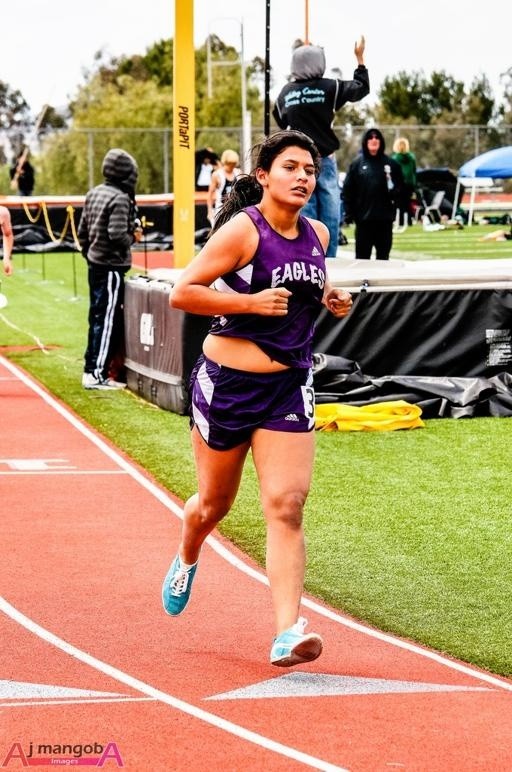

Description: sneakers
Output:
[161,553,198,617]
[270,616,323,666]
[84,373,126,390]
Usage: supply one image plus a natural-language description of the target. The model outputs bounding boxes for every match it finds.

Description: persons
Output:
[10,151,34,196]
[162,133,354,668]
[392,136,417,233]
[480,213,511,225]
[271,33,370,257]
[342,128,404,260]
[479,228,512,242]
[196,147,221,191]
[0,207,14,275]
[76,148,144,391]
[207,149,244,227]
